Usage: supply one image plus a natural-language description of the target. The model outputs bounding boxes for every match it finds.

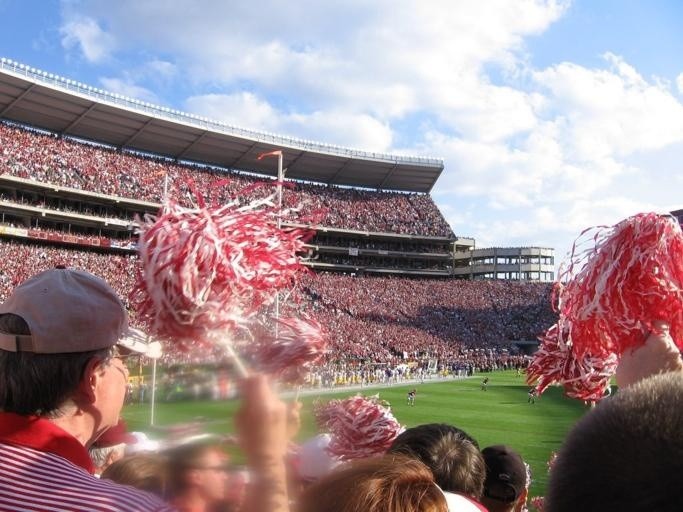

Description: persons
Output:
[1,264,173,511]
[1,237,565,404]
[529,317,683,511]
[0,118,456,238]
[90,371,529,511]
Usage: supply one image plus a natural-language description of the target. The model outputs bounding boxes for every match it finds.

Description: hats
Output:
[0,265,164,359]
[299,434,340,483]
[95,420,135,447]
[480,445,525,502]
